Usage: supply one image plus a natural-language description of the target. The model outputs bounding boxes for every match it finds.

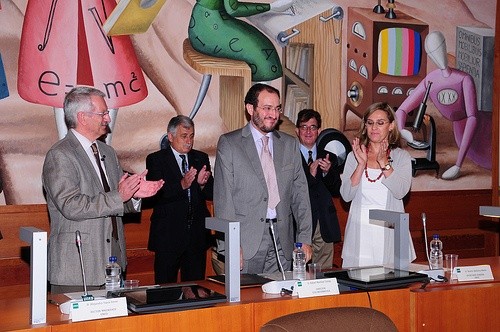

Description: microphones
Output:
[415,212,445,282]
[59,229,107,314]
[262,219,298,295]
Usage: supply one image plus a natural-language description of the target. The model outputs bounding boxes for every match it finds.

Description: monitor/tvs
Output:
[112,284,228,315]
[316,266,428,288]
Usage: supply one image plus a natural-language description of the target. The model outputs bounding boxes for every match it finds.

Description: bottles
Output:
[105,256,121,298]
[292,243,307,280]
[430,234,443,269]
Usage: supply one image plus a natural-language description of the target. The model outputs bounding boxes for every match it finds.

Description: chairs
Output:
[260,306,400,332]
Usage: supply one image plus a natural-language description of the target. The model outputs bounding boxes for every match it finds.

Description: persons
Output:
[41,87,165,294]
[145,115,214,285]
[340,102,416,268]
[291,109,342,272]
[214,83,312,276]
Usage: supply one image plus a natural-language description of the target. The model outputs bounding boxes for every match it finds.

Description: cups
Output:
[124,280,139,289]
[308,263,321,279]
[445,254,458,273]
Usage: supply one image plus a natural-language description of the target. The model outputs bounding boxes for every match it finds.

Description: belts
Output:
[266,218,277,224]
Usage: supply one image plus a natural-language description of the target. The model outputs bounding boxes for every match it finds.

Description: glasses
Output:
[298,124,318,131]
[252,104,284,115]
[82,109,110,119]
[365,120,389,126]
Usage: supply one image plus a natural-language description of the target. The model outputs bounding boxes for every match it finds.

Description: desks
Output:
[0,253,500,332]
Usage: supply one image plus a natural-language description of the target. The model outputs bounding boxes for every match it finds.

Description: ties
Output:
[261,135,281,210]
[179,154,191,203]
[91,142,119,244]
[307,150,313,167]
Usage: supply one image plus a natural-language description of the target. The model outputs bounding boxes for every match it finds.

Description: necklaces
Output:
[365,146,391,183]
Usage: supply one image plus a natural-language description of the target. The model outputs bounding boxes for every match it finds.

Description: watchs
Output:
[381,164,391,171]
